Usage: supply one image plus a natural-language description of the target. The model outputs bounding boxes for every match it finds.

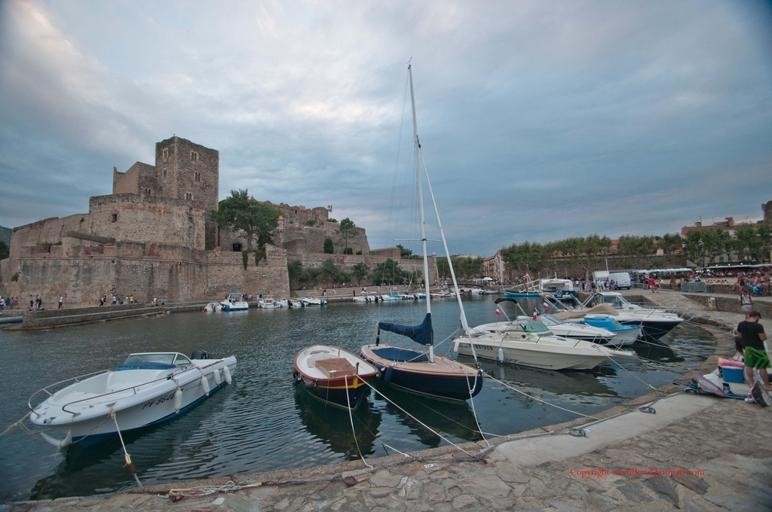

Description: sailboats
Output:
[363,65,483,403]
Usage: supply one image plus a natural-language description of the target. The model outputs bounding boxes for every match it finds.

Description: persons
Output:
[150,295,166,306]
[112,292,135,305]
[99,295,106,306]
[0,296,19,310]
[734,312,748,361]
[30,294,42,310]
[737,311,772,390]
[58,295,64,309]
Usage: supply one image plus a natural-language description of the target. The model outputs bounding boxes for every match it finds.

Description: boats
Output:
[296,344,378,409]
[353,287,487,303]
[28,350,237,439]
[457,288,684,372]
[220,292,322,311]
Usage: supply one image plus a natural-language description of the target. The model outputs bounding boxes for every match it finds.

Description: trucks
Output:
[593,271,608,288]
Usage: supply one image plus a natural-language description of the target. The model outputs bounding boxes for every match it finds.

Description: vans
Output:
[605,272,630,290]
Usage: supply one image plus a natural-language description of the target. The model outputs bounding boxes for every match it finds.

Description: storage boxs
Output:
[720,364,745,383]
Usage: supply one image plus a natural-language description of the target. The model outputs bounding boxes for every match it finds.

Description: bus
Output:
[645,269,693,285]
[703,264,772,285]
